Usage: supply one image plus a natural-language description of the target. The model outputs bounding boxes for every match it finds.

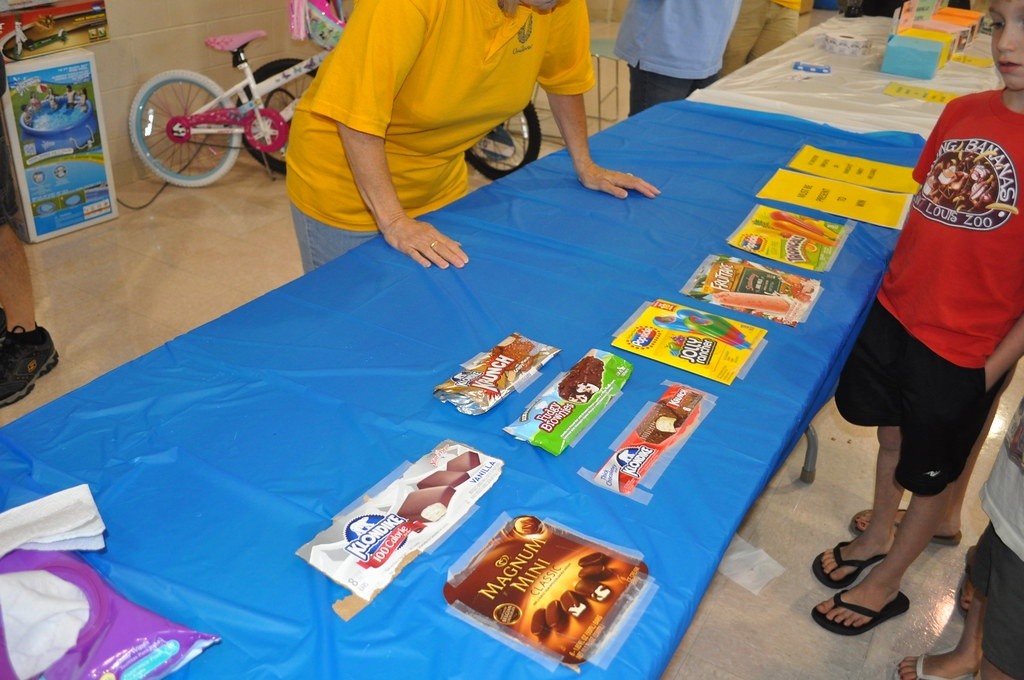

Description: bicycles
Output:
[126,0,349,188]
[238,0,543,176]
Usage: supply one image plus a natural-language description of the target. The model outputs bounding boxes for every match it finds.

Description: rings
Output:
[628,172,633,177]
[430,240,437,247]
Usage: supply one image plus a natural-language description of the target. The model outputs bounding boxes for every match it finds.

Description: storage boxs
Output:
[0,48,118,244]
[879,0,987,80]
[0,0,110,63]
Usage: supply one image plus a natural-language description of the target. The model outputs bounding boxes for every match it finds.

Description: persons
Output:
[717,0,801,78]
[286,0,660,275]
[20,84,88,127]
[848,358,1016,617]
[0,131,59,408]
[812,0,1023,636]
[613,0,742,118]
[894,397,1024,680]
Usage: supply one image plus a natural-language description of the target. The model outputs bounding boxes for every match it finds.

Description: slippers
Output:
[849,506,963,546]
[813,541,890,589]
[897,653,975,680]
[811,589,910,635]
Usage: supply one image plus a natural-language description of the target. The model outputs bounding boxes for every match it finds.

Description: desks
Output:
[0,100,929,680]
[685,13,1008,142]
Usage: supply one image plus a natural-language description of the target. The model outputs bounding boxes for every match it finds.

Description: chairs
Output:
[506,38,620,147]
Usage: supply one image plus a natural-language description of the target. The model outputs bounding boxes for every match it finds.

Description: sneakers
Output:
[0,323,60,409]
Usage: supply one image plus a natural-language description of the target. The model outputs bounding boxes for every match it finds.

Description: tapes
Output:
[824,29,872,59]
